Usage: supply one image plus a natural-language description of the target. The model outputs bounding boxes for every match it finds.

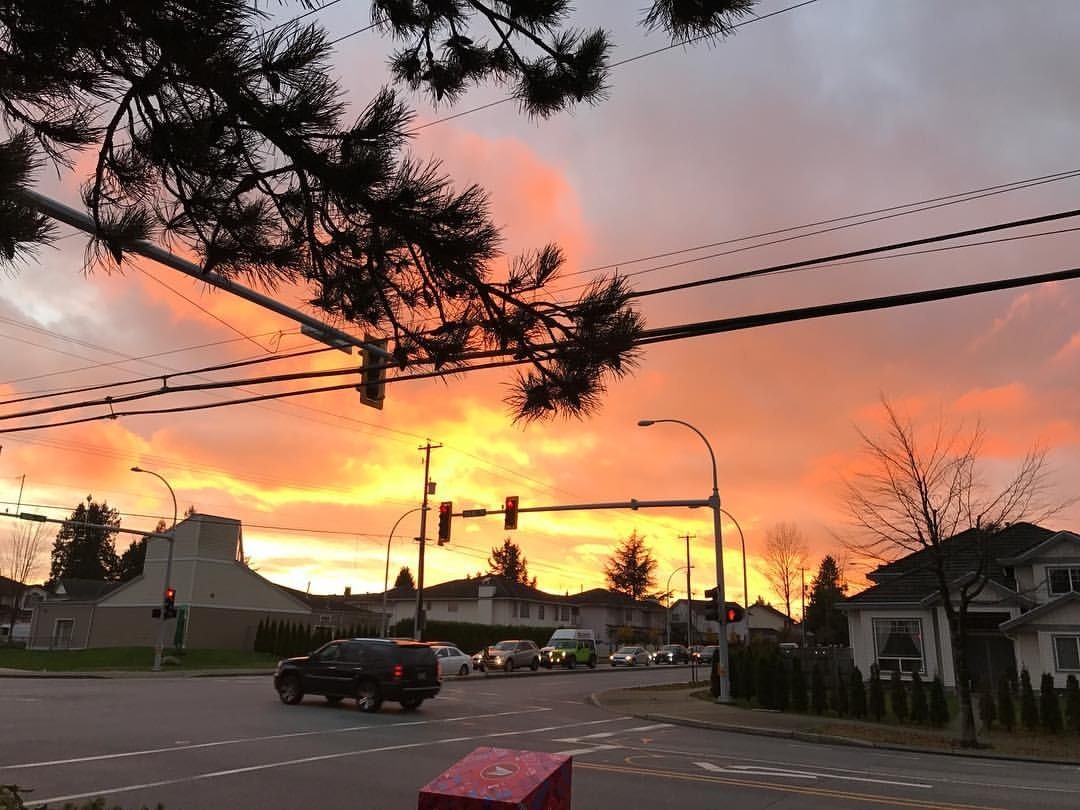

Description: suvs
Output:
[476,640,541,672]
[541,629,597,670]
[273,637,443,713]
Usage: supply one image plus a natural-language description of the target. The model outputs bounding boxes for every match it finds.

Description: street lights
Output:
[636,417,739,706]
[379,507,432,638]
[665,565,696,645]
[130,466,178,671]
[686,504,749,609]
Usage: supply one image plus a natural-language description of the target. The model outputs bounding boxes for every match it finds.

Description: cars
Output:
[429,645,475,675]
[472,645,495,671]
[653,644,689,664]
[610,647,653,667]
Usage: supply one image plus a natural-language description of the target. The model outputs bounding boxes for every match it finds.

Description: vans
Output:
[696,645,720,664]
[687,646,704,661]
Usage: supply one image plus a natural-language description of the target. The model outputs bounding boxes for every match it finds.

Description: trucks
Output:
[0,623,31,649]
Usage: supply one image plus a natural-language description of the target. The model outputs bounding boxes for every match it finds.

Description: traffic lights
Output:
[436,501,451,546]
[504,496,519,529]
[166,588,176,609]
[703,587,721,622]
[725,605,745,624]
